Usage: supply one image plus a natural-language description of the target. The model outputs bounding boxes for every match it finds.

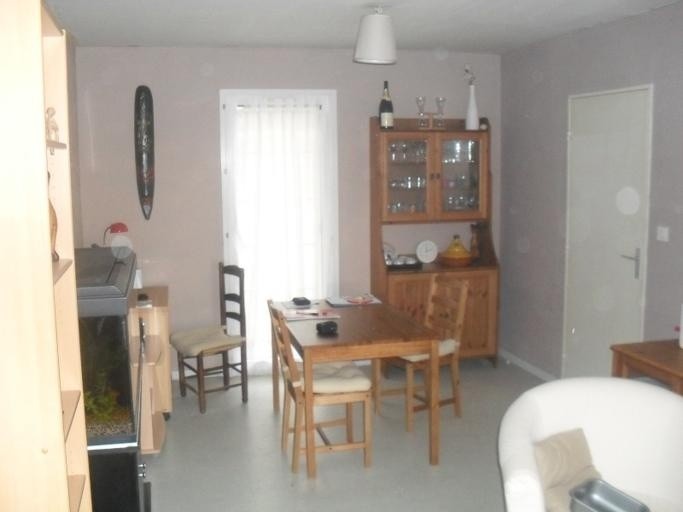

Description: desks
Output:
[272,293,440,479]
[608,338,683,395]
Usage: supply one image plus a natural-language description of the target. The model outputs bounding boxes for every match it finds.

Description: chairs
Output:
[499,377,681,512]
[371,271,463,432]
[176,262,249,414]
[265,298,374,473]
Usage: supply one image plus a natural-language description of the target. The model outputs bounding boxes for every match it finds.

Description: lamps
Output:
[102,223,129,246]
[353,0,397,66]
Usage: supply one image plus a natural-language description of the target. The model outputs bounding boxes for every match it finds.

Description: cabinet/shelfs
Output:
[370,115,502,379]
[126,284,172,455]
[0,0,94,512]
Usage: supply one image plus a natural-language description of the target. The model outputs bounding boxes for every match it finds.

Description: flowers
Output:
[464,68,476,85]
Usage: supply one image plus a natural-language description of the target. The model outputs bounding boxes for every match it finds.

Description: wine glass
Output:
[436,97,447,114]
[416,96,425,113]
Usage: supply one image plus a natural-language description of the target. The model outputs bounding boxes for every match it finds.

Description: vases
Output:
[465,84,480,131]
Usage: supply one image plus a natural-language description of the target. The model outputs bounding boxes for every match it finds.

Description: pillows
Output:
[534,429,597,510]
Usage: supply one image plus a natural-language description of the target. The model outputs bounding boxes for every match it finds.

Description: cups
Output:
[385,253,416,265]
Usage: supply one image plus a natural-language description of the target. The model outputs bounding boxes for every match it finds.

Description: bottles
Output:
[388,140,478,214]
[434,113,448,128]
[417,113,428,128]
[378,80,393,130]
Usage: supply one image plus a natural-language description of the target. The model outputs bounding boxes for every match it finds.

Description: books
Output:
[279,305,343,322]
[324,292,385,307]
